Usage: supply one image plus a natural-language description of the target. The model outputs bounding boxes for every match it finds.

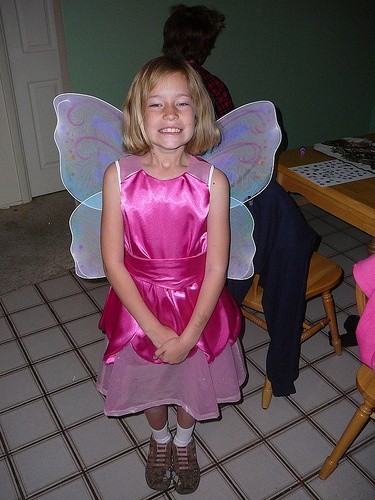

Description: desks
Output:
[273,131,375,256]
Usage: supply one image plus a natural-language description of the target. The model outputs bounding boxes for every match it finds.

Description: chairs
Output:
[235,250,343,409]
[319,257,375,481]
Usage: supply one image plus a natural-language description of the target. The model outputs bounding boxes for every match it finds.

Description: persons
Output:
[162,4,235,120]
[96,54,248,495]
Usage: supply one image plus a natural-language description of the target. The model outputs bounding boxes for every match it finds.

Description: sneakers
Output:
[172,436,200,494]
[145,431,172,491]
